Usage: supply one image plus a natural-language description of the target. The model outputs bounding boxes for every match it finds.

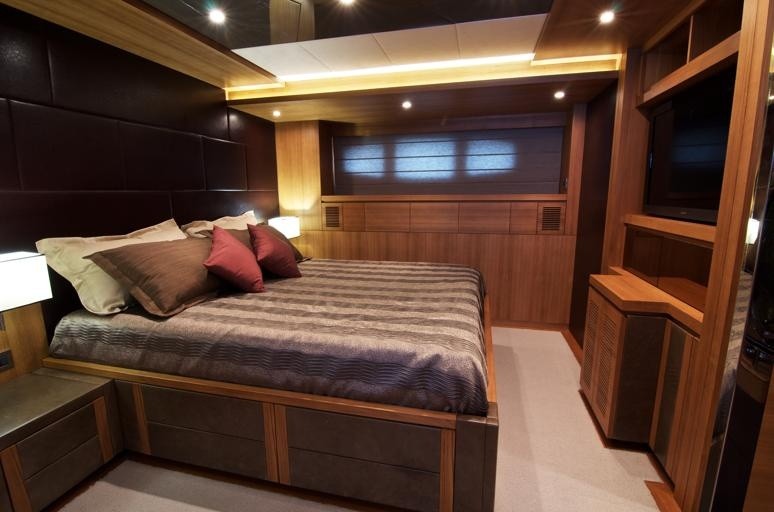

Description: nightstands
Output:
[0,367,125,512]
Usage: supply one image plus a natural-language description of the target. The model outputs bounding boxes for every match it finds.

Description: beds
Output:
[42,259,498,512]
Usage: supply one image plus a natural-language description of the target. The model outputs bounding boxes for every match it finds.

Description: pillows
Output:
[202,224,266,294]
[180,210,257,239]
[83,238,239,318]
[34,218,186,315]
[247,222,301,278]
[195,221,310,262]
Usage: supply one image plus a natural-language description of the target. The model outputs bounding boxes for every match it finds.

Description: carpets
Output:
[55,327,667,512]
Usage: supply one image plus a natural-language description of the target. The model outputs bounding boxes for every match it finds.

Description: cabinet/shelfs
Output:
[622,51,737,322]
[580,274,668,443]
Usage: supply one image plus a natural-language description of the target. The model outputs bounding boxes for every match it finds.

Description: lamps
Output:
[267,216,301,240]
[0,250,53,315]
